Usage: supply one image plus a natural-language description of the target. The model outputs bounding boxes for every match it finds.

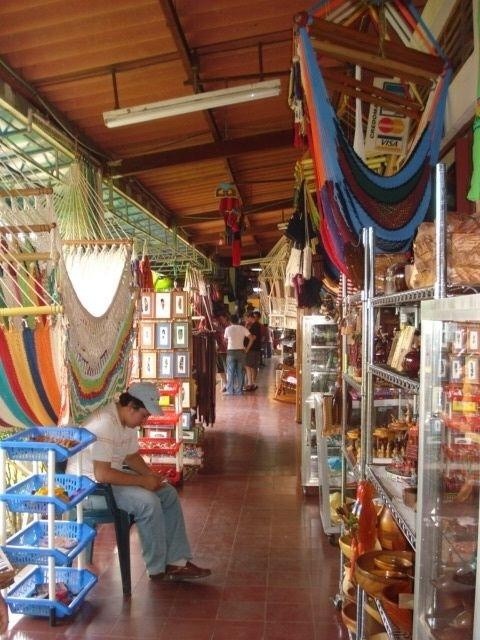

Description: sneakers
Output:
[242,384,258,392]
[148,573,168,581]
[220,386,227,392]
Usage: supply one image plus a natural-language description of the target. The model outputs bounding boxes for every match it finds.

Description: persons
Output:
[216,311,271,395]
[65,382,212,586]
[161,298,165,311]
[161,330,166,340]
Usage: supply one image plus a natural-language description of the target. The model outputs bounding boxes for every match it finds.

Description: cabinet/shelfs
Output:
[1,425,98,624]
[137,382,184,487]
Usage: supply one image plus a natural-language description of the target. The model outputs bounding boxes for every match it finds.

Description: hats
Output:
[126,381,166,417]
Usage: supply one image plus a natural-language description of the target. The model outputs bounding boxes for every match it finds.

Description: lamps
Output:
[103,80,280,128]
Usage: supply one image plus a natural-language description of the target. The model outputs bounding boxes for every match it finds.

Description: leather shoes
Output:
[165,560,212,579]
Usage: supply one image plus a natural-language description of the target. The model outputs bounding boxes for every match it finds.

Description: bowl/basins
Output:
[341,601,359,633]
[382,584,413,632]
[354,550,412,592]
[404,488,419,507]
[339,534,356,558]
[375,554,413,569]
